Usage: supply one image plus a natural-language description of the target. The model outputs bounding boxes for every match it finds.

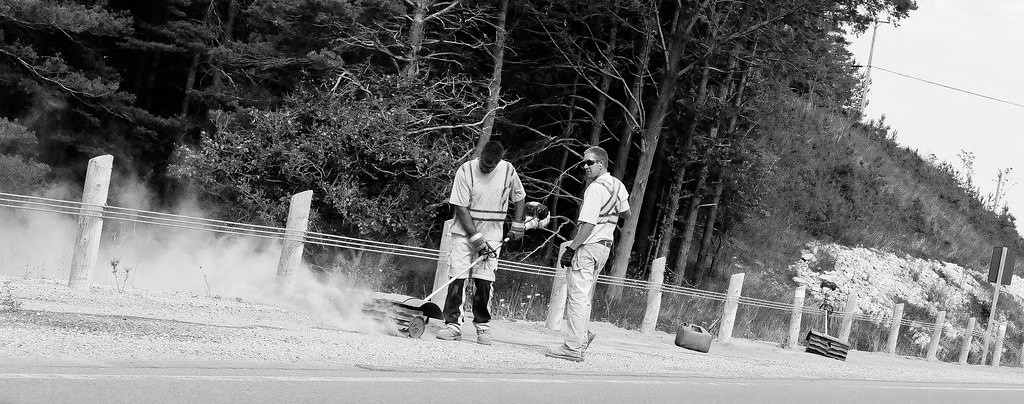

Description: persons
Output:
[435,140,527,345]
[545,147,632,360]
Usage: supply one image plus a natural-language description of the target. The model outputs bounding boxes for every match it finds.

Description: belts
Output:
[597,239,612,249]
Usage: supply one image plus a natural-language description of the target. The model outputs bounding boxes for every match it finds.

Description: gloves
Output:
[509,222,526,240]
[561,246,576,269]
[469,232,489,262]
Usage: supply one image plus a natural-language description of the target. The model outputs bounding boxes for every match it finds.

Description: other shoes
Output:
[546,343,586,362]
[473,322,491,345]
[436,323,461,341]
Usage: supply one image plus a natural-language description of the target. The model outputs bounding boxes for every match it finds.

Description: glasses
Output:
[582,160,602,167]
